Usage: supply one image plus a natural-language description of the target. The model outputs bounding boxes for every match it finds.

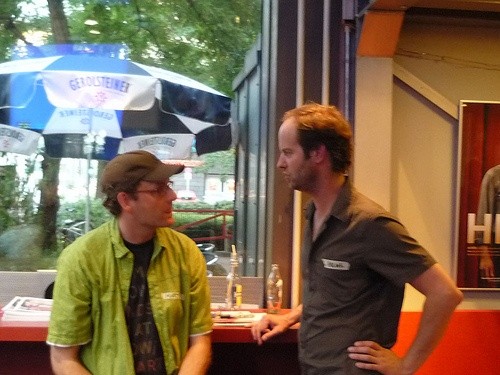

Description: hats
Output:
[101,150,185,193]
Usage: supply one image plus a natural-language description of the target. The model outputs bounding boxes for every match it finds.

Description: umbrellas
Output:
[0,55,232,234]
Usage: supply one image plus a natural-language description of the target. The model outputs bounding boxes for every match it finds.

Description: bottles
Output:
[266,264,283,313]
[226,254,243,309]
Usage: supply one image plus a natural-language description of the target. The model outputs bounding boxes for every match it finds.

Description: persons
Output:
[45,150,214,375]
[250,101,464,375]
[475,164,500,288]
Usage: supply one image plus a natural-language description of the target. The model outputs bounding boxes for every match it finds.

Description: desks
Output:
[0,305,302,344]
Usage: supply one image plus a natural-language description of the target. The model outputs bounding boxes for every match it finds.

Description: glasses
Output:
[127,181,174,196]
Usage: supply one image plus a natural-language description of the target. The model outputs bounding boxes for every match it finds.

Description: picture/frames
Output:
[454,100,500,291]
